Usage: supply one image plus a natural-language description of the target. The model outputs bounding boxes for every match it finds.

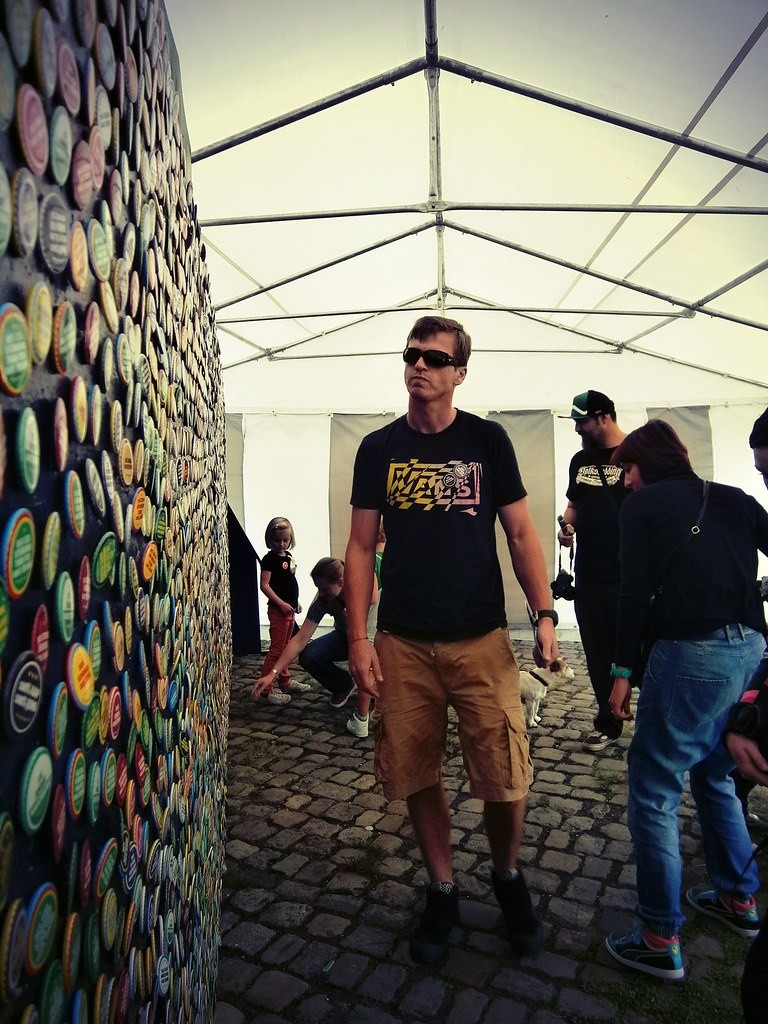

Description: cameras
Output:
[550,573,576,600]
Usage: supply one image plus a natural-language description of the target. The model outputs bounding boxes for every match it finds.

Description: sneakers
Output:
[686,888,759,937]
[283,681,310,694]
[346,710,369,738]
[604,930,684,978]
[582,729,618,752]
[259,685,291,705]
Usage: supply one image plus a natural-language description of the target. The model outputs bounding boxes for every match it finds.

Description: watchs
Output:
[610,663,633,678]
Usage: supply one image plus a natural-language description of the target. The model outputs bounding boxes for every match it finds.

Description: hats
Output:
[558,392,615,422]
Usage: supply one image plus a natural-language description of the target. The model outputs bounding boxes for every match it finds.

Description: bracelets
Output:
[272,669,279,675]
[530,610,558,627]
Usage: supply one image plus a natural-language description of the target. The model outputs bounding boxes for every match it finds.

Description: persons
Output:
[608,419,768,979]
[558,390,635,752]
[251,557,376,716]
[347,518,387,738]
[260,517,311,705]
[719,407,768,1024]
[343,316,560,966]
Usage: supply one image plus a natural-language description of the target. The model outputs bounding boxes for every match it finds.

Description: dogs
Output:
[519,658,575,727]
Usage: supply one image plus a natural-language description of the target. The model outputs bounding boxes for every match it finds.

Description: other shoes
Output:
[751,841,760,855]
[330,680,357,707]
[746,811,760,822]
[490,871,544,958]
[409,883,460,965]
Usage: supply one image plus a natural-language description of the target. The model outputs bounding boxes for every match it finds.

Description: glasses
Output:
[403,346,460,368]
